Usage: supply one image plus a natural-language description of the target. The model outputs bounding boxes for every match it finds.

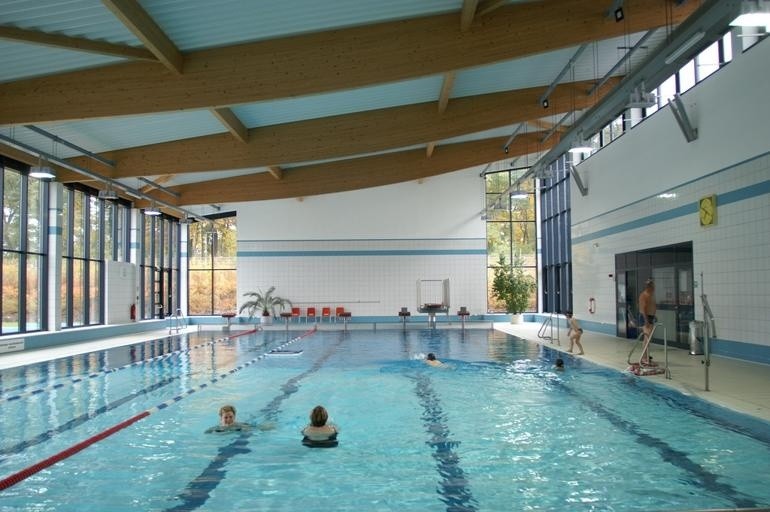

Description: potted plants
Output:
[238,287,292,326]
[492,249,536,323]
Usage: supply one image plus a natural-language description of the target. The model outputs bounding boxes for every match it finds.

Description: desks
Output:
[417,304,449,328]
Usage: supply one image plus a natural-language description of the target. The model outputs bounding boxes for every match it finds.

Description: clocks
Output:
[699,195,717,226]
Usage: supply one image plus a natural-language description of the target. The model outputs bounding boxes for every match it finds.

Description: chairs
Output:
[290,307,344,323]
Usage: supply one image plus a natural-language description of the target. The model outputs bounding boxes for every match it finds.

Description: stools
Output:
[338,312,351,328]
[281,313,291,328]
[222,313,235,327]
[399,307,410,328]
[456,307,470,327]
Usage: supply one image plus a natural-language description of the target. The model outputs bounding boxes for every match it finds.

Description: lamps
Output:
[510,156,529,200]
[28,154,55,180]
[568,58,594,155]
[97,178,217,234]
[727,0,770,29]
[663,31,706,65]
[622,1,656,109]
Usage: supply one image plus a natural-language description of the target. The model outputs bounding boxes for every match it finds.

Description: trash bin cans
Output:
[689,320,704,355]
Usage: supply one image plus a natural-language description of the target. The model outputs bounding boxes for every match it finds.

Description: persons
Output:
[565,311,584,355]
[301,405,339,448]
[426,353,457,369]
[204,406,276,436]
[639,280,657,363]
[555,359,564,370]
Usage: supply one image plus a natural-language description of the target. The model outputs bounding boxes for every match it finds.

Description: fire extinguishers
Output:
[589,298,595,313]
[131,304,136,320]
